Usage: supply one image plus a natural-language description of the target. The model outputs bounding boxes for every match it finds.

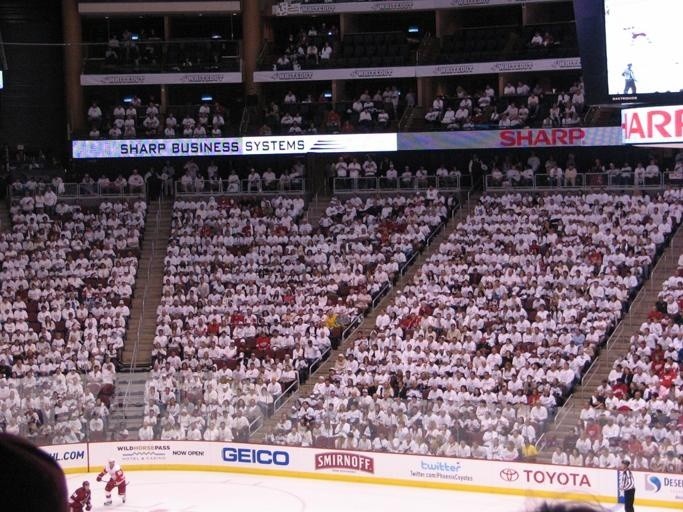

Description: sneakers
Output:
[104,501,112,505]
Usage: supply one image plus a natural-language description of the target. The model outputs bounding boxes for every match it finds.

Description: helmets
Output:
[83,480,90,489]
[108,457,116,462]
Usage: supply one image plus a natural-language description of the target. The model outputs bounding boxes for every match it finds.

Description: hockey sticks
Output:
[100,480,130,486]
